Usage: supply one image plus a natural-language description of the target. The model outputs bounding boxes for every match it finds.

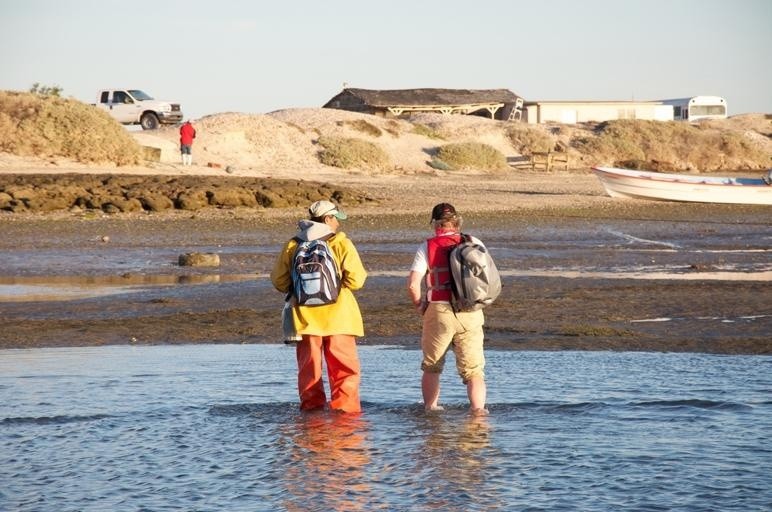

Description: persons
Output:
[180,120,196,166]
[407,203,488,412]
[269,199,368,414]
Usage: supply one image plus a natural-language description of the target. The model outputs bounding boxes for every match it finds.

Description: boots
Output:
[182,155,192,166]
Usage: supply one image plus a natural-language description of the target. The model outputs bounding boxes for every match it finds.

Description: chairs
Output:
[507,98,528,121]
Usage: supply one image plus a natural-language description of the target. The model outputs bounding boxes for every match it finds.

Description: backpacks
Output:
[448,233,503,311]
[289,231,345,308]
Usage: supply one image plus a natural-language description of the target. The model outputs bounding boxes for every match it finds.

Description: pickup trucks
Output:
[90,88,184,130]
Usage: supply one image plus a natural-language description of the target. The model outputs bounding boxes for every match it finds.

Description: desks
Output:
[532,150,568,173]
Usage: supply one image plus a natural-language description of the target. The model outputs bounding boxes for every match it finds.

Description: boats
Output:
[589,165,772,204]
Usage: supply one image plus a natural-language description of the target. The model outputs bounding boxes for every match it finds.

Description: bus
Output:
[650,95,729,122]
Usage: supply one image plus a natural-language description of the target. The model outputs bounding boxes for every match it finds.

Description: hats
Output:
[309,198,347,222]
[187,118,196,123]
[428,201,456,226]
